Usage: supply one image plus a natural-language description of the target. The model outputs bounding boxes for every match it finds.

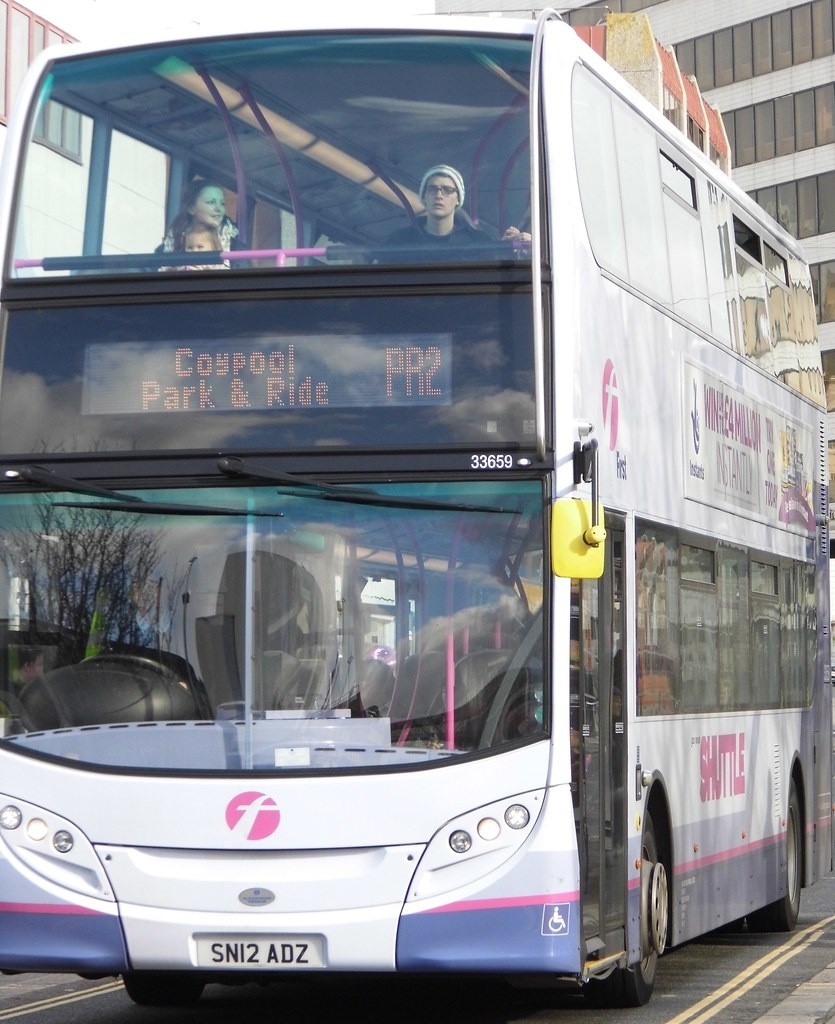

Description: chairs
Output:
[423,649,531,741]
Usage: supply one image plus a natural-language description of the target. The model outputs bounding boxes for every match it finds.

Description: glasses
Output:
[424,185,457,195]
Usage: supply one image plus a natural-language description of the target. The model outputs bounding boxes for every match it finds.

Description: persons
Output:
[386,163,491,243]
[156,178,242,255]
[4,646,45,710]
[155,223,233,271]
[62,574,185,663]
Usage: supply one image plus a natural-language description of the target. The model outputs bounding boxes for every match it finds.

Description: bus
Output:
[1,6,835,1012]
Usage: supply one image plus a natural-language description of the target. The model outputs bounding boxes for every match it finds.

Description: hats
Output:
[418,168,464,208]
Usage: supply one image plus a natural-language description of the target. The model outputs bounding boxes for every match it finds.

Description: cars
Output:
[362,642,397,676]
